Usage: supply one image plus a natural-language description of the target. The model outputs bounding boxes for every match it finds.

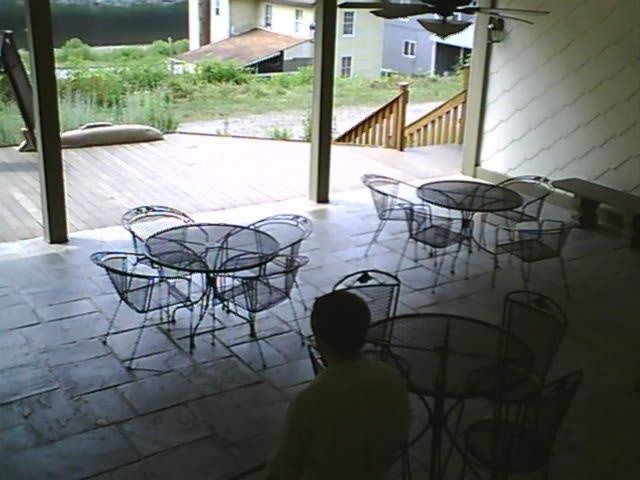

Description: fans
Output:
[337,0,550,36]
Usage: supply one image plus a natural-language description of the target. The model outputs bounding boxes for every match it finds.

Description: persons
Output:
[263,290,412,479]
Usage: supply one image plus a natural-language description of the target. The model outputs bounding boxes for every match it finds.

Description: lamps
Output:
[485,8,508,45]
[417,14,471,38]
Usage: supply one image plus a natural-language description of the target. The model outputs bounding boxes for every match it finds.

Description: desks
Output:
[416,180,524,273]
[145,223,281,346]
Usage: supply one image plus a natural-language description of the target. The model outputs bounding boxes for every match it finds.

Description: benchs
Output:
[552,177,639,248]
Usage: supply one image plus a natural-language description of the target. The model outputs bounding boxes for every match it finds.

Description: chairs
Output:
[477,175,554,250]
[360,174,433,263]
[214,213,314,315]
[91,251,192,370]
[492,219,575,306]
[121,206,209,311]
[307,269,584,478]
[209,255,310,367]
[393,203,474,292]
